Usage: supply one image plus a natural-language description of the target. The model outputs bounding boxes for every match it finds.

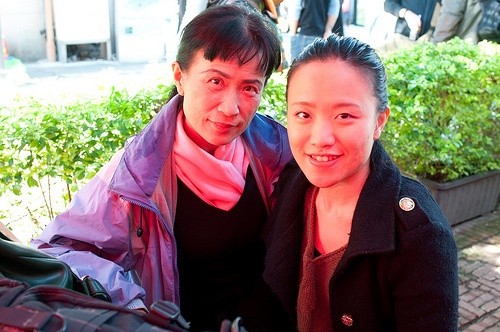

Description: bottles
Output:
[409,14,422,42]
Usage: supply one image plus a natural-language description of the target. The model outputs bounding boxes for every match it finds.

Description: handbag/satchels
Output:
[0,238,191,332]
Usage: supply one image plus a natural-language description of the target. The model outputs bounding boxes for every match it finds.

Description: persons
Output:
[28,4,293,332]
[259,33,459,332]
[206,1,500,78]
